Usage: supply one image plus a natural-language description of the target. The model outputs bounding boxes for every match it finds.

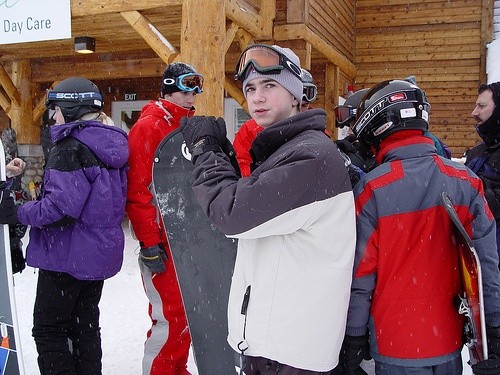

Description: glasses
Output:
[335,106,357,126]
[176,72,203,94]
[234,45,300,80]
[44,89,101,101]
[301,82,317,101]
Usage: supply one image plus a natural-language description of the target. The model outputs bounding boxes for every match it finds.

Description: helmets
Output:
[351,80,428,145]
[48,77,103,123]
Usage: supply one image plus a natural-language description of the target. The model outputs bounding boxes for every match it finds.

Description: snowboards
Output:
[152,124,241,375]
[0,139,27,375]
[441,189,500,374]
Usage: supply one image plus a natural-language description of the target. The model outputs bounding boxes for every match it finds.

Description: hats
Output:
[242,46,303,102]
[162,62,197,93]
[301,68,315,84]
[344,89,369,107]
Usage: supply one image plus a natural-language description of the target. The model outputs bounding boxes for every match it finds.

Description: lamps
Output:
[74,36,96,54]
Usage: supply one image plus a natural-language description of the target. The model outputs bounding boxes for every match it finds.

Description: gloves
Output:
[340,332,371,368]
[139,247,166,272]
[181,115,226,156]
[472,329,500,375]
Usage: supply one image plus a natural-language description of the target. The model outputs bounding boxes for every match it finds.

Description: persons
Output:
[0,77,129,375]
[179,42,357,375]
[344,79,500,375]
[124,62,205,375]
[231,68,500,375]
